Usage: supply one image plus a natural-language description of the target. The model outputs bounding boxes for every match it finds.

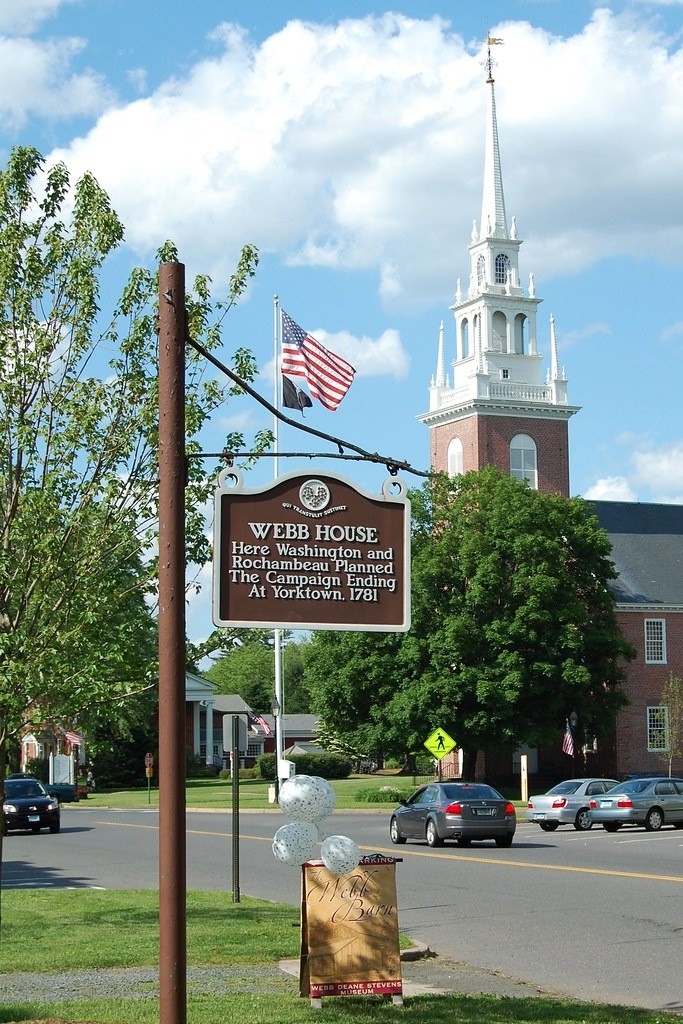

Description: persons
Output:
[86,768,93,793]
[24,784,36,797]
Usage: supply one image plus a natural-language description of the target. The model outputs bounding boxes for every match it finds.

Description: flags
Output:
[279,377,312,416]
[279,307,354,413]
[563,722,576,758]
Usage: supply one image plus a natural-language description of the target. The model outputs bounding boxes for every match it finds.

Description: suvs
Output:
[0,776,62,836]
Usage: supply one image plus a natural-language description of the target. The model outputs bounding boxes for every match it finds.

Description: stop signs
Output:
[144,752,155,769]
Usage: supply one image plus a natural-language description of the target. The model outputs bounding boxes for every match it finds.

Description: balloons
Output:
[272,774,360,875]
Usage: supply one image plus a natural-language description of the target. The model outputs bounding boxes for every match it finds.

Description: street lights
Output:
[272,695,281,806]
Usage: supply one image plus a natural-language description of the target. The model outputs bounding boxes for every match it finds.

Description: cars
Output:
[389,782,517,848]
[588,776,682,831]
[526,778,623,831]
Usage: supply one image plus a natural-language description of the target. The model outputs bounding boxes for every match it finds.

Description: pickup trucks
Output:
[5,772,79,805]
